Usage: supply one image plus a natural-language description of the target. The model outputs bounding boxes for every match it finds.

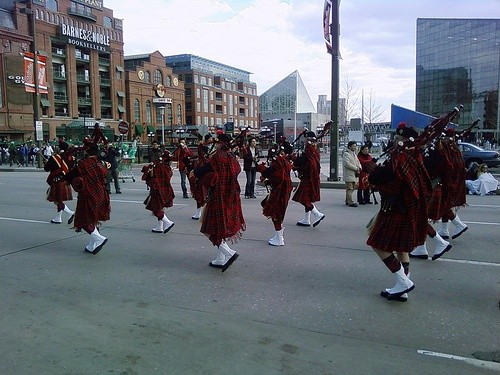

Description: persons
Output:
[255,143,293,246]
[62,134,112,255]
[288,131,326,228]
[173,138,195,198]
[467,161,500,196]
[357,145,376,204]
[0,141,55,168]
[243,136,260,199]
[140,143,176,234]
[204,133,218,156]
[265,144,278,165]
[148,140,163,162]
[341,140,362,208]
[42,136,76,224]
[366,122,468,302]
[99,140,122,194]
[182,130,246,273]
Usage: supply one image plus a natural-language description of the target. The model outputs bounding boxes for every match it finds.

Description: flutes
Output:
[297,119,336,160]
[361,102,487,189]
[37,150,51,165]
[196,135,217,165]
[76,121,112,169]
[204,123,253,168]
[152,142,182,168]
[257,128,311,185]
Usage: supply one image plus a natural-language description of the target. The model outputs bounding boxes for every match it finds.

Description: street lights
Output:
[157,106,167,146]
[19,7,43,168]
[272,122,278,143]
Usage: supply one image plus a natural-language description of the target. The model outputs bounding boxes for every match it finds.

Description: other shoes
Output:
[116,190,121,194]
[346,202,357,205]
[349,204,358,207]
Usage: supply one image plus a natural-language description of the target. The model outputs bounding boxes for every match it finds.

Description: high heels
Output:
[245,194,256,199]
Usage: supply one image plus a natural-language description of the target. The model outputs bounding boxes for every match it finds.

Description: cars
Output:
[456,142,500,170]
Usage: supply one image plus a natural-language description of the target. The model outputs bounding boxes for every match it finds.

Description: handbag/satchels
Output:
[355,167,369,190]
[354,177,359,190]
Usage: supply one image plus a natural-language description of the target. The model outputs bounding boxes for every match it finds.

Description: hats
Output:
[304,131,315,137]
[396,122,418,141]
[59,138,69,149]
[213,134,233,143]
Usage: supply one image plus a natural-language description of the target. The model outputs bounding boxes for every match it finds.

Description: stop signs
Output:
[118,121,129,135]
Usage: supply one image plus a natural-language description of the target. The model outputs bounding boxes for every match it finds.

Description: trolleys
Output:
[110,157,136,183]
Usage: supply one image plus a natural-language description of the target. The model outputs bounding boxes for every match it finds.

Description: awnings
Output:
[118,91,125,97]
[136,125,155,133]
[116,66,125,72]
[118,107,126,112]
[41,100,51,106]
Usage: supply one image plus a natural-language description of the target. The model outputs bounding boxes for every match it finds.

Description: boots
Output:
[311,205,325,227]
[409,241,428,260]
[162,214,174,234]
[192,208,201,220]
[450,213,468,239]
[386,269,415,301]
[152,220,163,233]
[50,210,62,224]
[438,223,449,239]
[64,204,75,224]
[85,227,108,255]
[431,231,452,261]
[297,211,311,227]
[380,272,411,302]
[268,229,285,246]
[209,240,239,272]
